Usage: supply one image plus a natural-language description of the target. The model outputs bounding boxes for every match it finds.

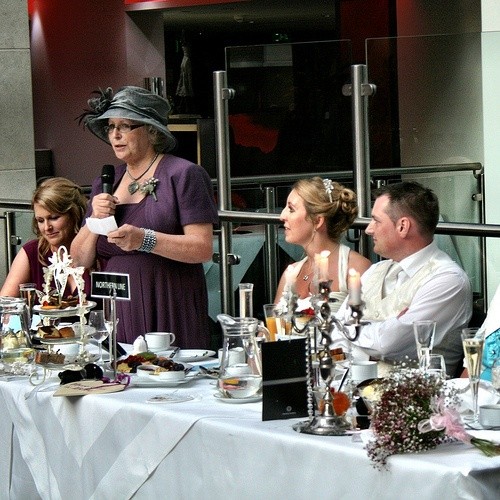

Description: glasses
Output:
[102,123,146,134]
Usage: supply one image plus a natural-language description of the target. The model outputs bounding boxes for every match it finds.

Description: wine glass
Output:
[237,283,254,375]
[88,310,108,368]
[460,327,486,419]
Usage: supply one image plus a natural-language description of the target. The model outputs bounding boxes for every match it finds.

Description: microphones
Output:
[101,165,116,194]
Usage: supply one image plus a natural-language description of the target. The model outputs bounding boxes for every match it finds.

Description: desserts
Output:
[36,297,79,365]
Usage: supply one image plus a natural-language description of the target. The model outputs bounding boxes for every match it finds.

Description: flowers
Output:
[364,354,500,472]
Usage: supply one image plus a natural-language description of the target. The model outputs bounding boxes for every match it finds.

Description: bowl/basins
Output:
[225,362,248,376]
[222,375,262,398]
[136,365,185,381]
[50,342,80,361]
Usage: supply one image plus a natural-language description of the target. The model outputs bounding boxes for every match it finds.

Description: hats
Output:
[85,86,176,153]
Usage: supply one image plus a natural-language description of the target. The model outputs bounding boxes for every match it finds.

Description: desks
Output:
[0,357,500,500]
[201,228,354,322]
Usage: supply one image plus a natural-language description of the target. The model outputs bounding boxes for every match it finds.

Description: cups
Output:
[477,405,500,427]
[349,359,378,384]
[413,319,446,384]
[145,332,175,349]
[19,283,37,338]
[264,304,292,341]
[217,347,246,367]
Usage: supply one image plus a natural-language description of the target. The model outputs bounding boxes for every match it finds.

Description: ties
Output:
[382,262,403,299]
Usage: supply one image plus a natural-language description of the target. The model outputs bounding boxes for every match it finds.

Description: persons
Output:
[0,177,97,333]
[459,284,500,392]
[70,86,215,349]
[322,181,474,380]
[247,177,372,372]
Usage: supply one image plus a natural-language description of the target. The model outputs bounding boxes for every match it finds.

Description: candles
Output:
[349,271,361,305]
[286,270,296,288]
[315,256,329,280]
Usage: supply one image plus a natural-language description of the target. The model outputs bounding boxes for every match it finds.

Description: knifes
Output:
[169,347,179,358]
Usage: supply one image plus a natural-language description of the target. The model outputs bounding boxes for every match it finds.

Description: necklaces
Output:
[302,245,338,281]
[125,153,159,196]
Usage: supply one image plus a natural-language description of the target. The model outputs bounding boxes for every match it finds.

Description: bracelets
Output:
[137,228,156,252]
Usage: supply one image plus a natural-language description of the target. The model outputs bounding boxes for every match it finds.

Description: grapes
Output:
[137,351,158,366]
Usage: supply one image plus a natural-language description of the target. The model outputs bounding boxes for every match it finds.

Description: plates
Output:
[35,325,96,345]
[183,357,218,366]
[104,361,194,386]
[32,301,96,316]
[213,393,261,403]
[468,420,500,429]
[438,377,499,425]
[38,363,66,370]
[149,347,215,361]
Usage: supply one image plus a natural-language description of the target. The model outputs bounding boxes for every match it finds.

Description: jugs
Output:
[0,297,34,382]
[216,313,270,398]
